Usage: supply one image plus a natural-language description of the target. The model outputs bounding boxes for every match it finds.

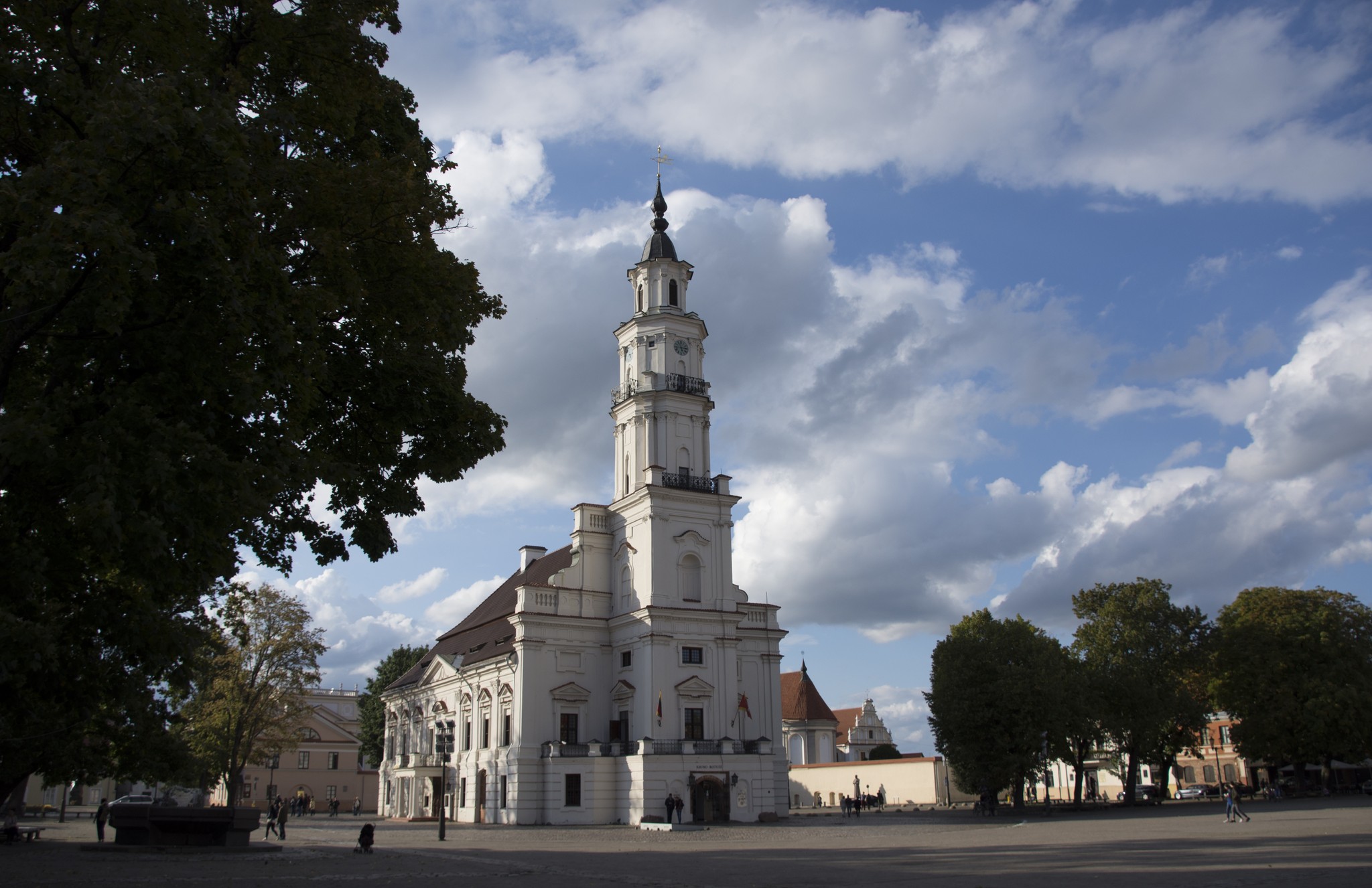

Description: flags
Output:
[738,694,752,720]
[656,691,662,720]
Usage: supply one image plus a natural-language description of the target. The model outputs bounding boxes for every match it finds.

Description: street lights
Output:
[434,720,456,841]
[1208,736,1226,801]
[268,755,280,815]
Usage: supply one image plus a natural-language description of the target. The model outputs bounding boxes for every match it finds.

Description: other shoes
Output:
[263,838,267,841]
[275,836,279,841]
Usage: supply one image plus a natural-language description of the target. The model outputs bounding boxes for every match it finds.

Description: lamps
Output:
[731,773,738,786]
[687,773,695,786]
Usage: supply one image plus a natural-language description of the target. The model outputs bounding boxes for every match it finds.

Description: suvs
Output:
[1116,783,1158,804]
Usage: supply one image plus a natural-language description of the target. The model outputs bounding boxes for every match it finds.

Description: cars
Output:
[106,794,154,810]
[1198,781,1254,797]
[1174,783,1213,799]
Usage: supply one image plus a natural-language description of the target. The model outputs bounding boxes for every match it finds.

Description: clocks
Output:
[625,347,633,363]
[674,339,689,355]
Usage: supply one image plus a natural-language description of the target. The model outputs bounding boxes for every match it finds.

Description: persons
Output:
[93,798,108,842]
[4,810,20,845]
[840,795,861,817]
[665,793,675,823]
[675,796,684,824]
[290,793,315,817]
[353,796,360,815]
[1260,778,1281,802]
[862,792,871,810]
[328,797,339,817]
[978,792,995,817]
[818,796,822,807]
[853,775,860,800]
[273,795,282,816]
[262,804,280,841]
[1223,781,1250,822]
[277,802,288,839]
[877,790,884,810]
[1027,784,1037,803]
